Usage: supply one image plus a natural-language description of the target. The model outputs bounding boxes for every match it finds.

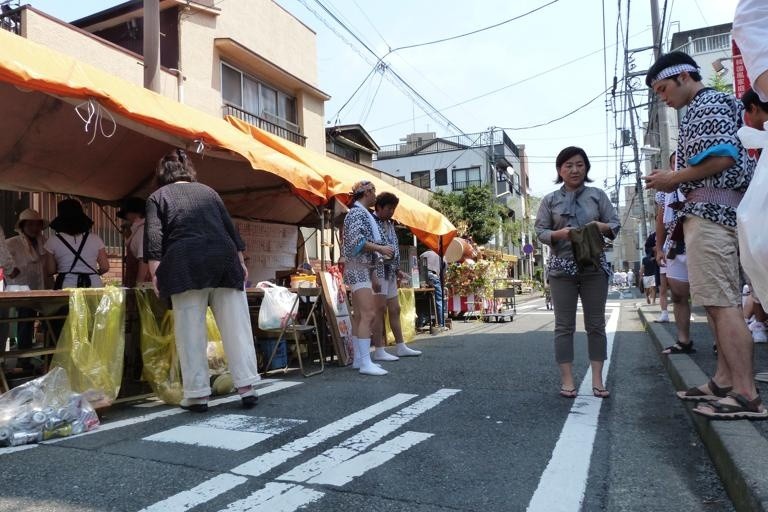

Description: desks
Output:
[2,284,164,411]
[241,287,302,310]
[396,286,440,330]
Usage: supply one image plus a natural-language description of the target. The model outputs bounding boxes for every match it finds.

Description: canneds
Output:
[0,394,98,446]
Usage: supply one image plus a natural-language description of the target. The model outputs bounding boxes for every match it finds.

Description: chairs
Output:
[252,284,329,380]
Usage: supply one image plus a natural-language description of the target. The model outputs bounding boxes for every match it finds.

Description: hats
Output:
[47,198,95,237]
[14,208,50,231]
[115,196,146,221]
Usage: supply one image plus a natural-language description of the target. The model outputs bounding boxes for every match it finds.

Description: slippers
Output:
[559,387,577,399]
[591,386,610,399]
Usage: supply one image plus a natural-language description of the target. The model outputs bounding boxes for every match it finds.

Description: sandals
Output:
[659,339,697,355]
[674,376,767,422]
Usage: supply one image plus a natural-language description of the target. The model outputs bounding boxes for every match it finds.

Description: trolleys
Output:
[482,278,517,323]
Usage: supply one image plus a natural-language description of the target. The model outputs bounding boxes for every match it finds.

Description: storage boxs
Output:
[256,337,288,370]
[289,274,317,289]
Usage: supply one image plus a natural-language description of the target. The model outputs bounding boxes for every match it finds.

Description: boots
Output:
[350,334,388,377]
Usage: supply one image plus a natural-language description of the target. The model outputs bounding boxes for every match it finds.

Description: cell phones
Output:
[644,173,655,183]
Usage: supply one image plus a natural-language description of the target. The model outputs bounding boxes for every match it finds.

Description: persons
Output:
[419,247,447,328]
[542,279,553,310]
[143,148,260,413]
[371,191,423,360]
[37,199,110,377]
[343,180,388,376]
[535,145,621,398]
[115,194,171,384]
[0,228,21,383]
[6,209,56,378]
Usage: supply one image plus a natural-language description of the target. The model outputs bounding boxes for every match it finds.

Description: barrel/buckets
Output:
[445,237,478,265]
[256,332,288,371]
[288,340,312,368]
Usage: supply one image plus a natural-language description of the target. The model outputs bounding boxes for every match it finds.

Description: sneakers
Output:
[652,310,670,324]
[395,342,422,357]
[239,387,258,407]
[372,346,400,362]
[178,397,208,414]
[749,321,767,344]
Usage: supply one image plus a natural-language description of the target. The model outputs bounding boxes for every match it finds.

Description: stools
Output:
[464,301,483,322]
